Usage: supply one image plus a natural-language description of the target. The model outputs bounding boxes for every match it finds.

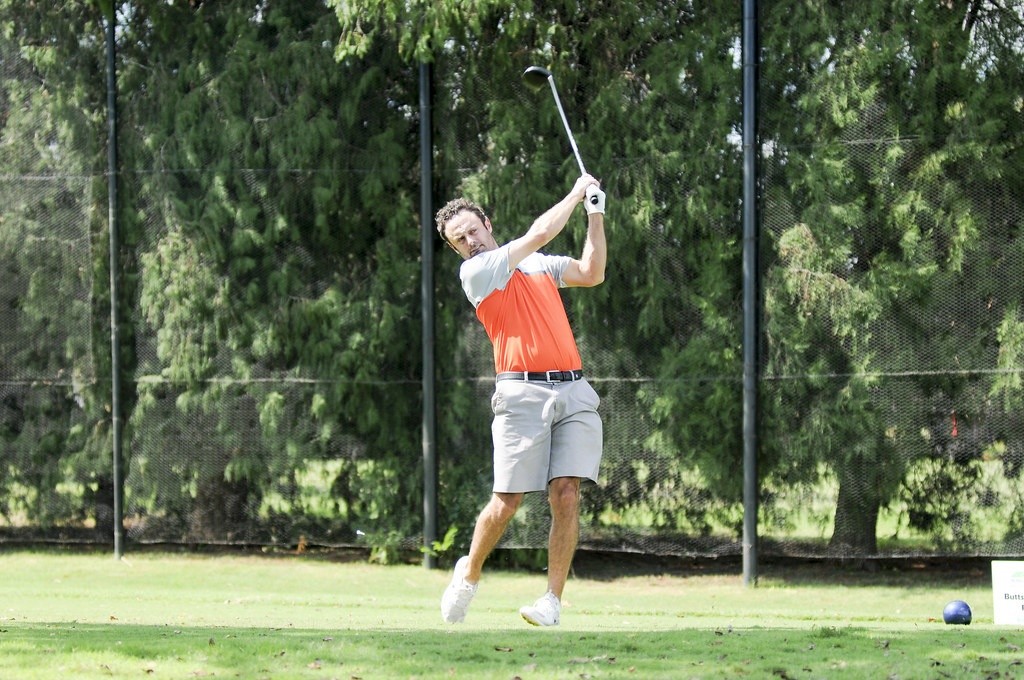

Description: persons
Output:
[434,173,607,627]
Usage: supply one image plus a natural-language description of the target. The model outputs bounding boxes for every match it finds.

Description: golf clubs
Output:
[522,64,600,205]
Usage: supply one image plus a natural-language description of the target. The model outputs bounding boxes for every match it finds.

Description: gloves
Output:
[583,185,606,216]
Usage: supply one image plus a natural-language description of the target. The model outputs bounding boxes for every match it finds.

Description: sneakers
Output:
[440,555,480,625]
[519,589,561,626]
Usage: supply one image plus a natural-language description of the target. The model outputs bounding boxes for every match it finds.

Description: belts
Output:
[496,370,583,383]
